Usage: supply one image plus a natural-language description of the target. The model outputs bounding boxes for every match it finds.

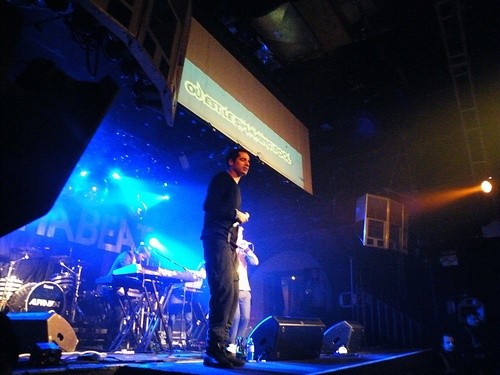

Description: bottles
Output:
[235,337,245,360]
[247,338,254,360]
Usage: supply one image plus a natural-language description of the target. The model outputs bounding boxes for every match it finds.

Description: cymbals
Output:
[9,247,40,258]
[47,255,73,262]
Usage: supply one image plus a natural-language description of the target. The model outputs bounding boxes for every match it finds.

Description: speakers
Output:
[6,311,80,357]
[247,314,326,361]
[321,320,366,353]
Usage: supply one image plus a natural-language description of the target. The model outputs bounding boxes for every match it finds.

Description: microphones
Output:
[140,241,153,249]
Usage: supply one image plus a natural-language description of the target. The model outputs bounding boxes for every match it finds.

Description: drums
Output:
[10,282,65,314]
[0,276,23,306]
[50,273,73,289]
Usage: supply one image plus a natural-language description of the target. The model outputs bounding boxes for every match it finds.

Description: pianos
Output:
[95,264,198,352]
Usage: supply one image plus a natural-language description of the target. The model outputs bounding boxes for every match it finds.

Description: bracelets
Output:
[244,248,250,254]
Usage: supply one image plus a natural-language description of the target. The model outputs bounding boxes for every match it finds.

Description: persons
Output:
[201,149,250,370]
[227,226,258,353]
[101,246,149,351]
[435,334,459,375]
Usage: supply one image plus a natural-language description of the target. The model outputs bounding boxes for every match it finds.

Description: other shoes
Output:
[224,349,245,367]
[204,348,232,368]
